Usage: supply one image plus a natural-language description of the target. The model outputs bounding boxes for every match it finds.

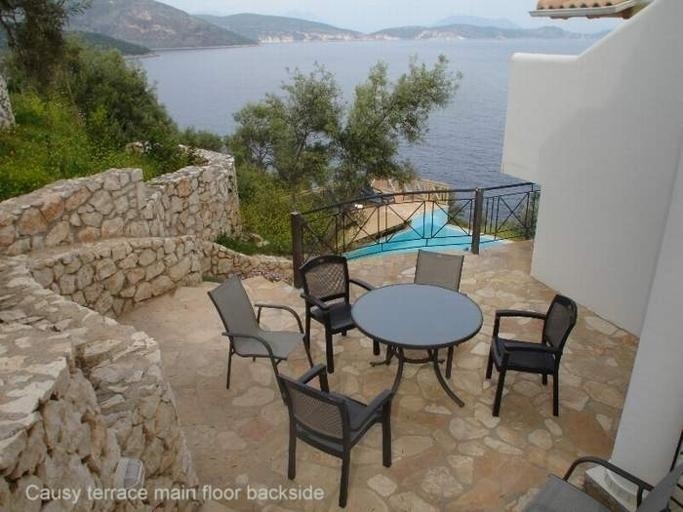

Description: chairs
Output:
[206,247,682,512]
